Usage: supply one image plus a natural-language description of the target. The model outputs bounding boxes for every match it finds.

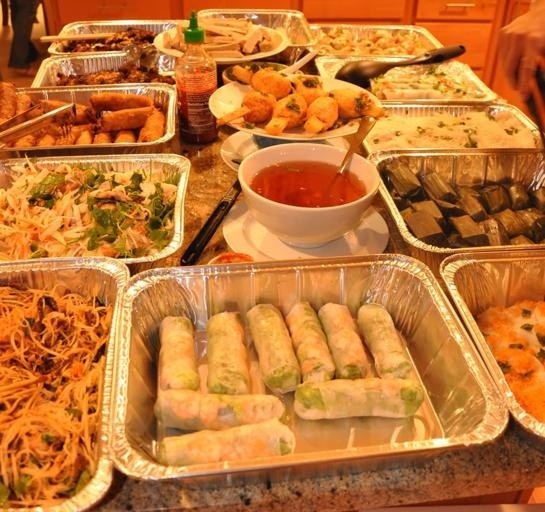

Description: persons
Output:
[496,2,544,100]
[7,0,47,77]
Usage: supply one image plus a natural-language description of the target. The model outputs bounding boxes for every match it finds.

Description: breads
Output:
[387,163,545,247]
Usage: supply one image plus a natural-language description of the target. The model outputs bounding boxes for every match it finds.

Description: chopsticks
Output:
[179,178,241,266]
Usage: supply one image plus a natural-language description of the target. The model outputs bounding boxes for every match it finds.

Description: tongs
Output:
[0,101,76,141]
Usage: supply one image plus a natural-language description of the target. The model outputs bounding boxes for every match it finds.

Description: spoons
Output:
[336,42,469,99]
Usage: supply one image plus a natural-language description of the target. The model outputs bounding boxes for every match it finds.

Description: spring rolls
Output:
[1,81,166,150]
[292,378,424,422]
[318,301,368,378]
[157,419,296,468]
[245,303,302,394]
[207,311,250,395]
[357,300,413,379]
[158,314,200,391]
[285,300,338,381]
[153,392,286,431]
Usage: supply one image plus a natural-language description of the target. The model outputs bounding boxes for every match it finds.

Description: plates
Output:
[367,149,545,278]
[106,251,511,486]
[437,251,545,449]
[361,103,544,150]
[316,51,502,108]
[31,53,176,88]
[0,83,176,161]
[1,258,114,511]
[206,68,382,141]
[151,21,289,65]
[217,196,391,263]
[46,19,191,54]
[197,11,316,66]
[2,152,192,275]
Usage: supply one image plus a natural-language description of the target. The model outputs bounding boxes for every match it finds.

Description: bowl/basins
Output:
[240,142,382,245]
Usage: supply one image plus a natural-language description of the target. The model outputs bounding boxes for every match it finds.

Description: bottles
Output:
[175,10,221,145]
